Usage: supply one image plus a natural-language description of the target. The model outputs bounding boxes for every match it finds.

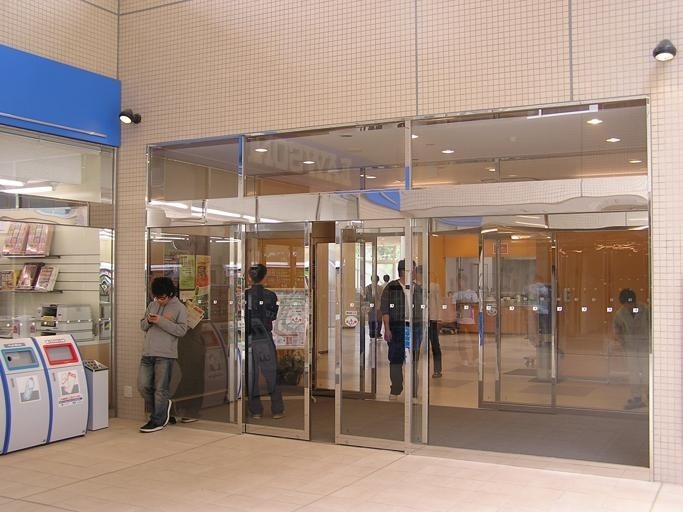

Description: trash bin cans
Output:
[83,359,109,431]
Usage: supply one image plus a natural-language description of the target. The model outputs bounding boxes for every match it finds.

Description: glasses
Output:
[154,295,168,300]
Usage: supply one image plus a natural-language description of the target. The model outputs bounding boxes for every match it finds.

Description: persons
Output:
[610,287,650,409]
[242,263,289,420]
[533,275,566,363]
[364,258,463,401]
[136,276,188,433]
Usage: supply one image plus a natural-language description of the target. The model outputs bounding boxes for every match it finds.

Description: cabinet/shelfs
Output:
[0,252,65,294]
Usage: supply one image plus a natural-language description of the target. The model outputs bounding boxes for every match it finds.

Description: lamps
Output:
[651,39,680,64]
[116,108,142,127]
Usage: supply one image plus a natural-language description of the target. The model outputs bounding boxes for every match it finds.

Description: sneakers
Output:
[162,399,172,427]
[432,371,442,377]
[272,410,287,418]
[413,398,418,404]
[182,417,197,423]
[389,393,396,400]
[139,422,163,432]
